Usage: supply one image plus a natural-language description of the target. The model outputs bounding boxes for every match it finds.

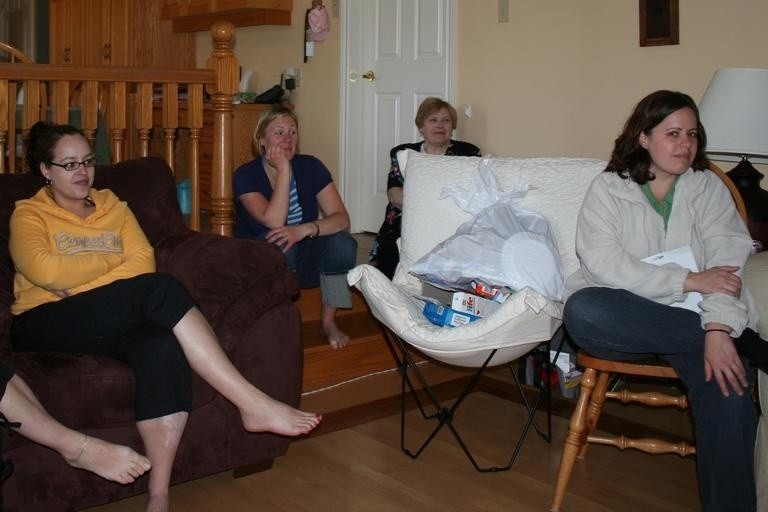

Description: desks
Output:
[5,102,116,170]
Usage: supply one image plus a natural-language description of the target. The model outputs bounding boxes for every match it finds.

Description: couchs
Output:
[739,244,767,512]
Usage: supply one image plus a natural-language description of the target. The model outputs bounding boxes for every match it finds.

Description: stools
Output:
[544,347,698,512]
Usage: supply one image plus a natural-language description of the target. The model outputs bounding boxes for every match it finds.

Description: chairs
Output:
[337,148,610,473]
[0,42,49,174]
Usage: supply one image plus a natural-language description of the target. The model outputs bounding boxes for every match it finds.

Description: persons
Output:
[370,97,482,280]
[564,89,768,510]
[0,363,151,485]
[233,107,357,349]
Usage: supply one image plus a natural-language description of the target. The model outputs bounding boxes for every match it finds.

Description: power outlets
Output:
[280,66,303,92]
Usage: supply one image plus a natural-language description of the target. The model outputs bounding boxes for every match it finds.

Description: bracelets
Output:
[705,329,731,336]
[311,221,320,239]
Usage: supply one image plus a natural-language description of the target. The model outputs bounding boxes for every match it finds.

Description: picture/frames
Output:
[637,0,680,48]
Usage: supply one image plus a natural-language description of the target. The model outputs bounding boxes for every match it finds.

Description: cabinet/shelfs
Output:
[139,97,276,229]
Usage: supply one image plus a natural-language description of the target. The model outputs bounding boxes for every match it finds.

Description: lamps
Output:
[693,66,767,251]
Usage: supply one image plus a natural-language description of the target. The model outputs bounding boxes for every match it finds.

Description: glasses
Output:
[48,156,100,172]
